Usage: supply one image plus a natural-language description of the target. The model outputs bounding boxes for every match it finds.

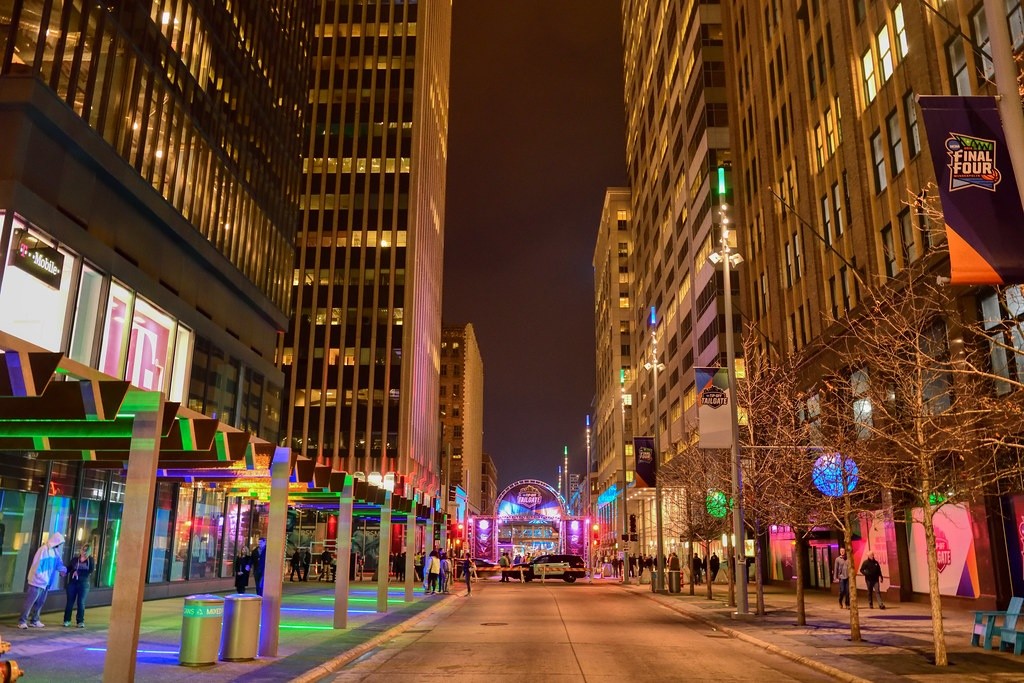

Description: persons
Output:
[860,552,885,609]
[833,547,850,610]
[593,552,624,578]
[350,549,361,580]
[693,552,719,586]
[235,538,267,597]
[463,553,471,597]
[317,547,337,583]
[500,552,536,583]
[17,532,67,630]
[62,543,95,628]
[290,548,311,582]
[664,552,681,571]
[726,556,751,583]
[414,547,463,596]
[389,551,406,581]
[629,553,658,578]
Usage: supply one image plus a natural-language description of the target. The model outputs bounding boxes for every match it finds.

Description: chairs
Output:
[969,597,1024,656]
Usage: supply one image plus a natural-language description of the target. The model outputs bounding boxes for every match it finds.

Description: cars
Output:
[471,558,500,567]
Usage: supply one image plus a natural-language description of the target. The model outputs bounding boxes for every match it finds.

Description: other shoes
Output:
[426,591,431,594]
[438,591,441,592]
[870,606,873,609]
[846,606,850,610]
[319,578,321,582]
[499,580,511,582]
[299,579,302,582]
[290,580,294,582]
[432,592,435,595]
[840,606,843,609]
[303,580,307,582]
[464,594,472,597]
[326,580,330,582]
[879,604,885,609]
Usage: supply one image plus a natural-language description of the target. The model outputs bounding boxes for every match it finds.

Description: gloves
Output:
[880,576,883,583]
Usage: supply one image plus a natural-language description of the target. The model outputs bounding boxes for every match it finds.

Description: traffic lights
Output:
[622,534,629,542]
[629,515,637,541]
[593,524,600,545]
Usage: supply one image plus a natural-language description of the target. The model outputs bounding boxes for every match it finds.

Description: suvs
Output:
[509,554,586,583]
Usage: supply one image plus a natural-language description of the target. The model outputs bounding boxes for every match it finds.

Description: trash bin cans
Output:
[669,571,681,593]
[224,593,263,661]
[651,571,664,593]
[180,593,225,666]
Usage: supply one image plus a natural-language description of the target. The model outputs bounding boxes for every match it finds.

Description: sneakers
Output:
[77,623,85,628]
[63,621,71,627]
[17,623,28,630]
[29,621,45,628]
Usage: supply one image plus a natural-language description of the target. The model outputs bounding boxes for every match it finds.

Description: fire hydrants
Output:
[0,635,24,683]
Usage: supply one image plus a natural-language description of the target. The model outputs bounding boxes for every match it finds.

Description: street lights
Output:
[618,369,633,583]
[564,445,568,502]
[584,414,594,576]
[705,166,754,619]
[558,466,561,493]
[643,307,667,594]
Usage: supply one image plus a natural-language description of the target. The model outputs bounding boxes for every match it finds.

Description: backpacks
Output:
[467,560,476,573]
